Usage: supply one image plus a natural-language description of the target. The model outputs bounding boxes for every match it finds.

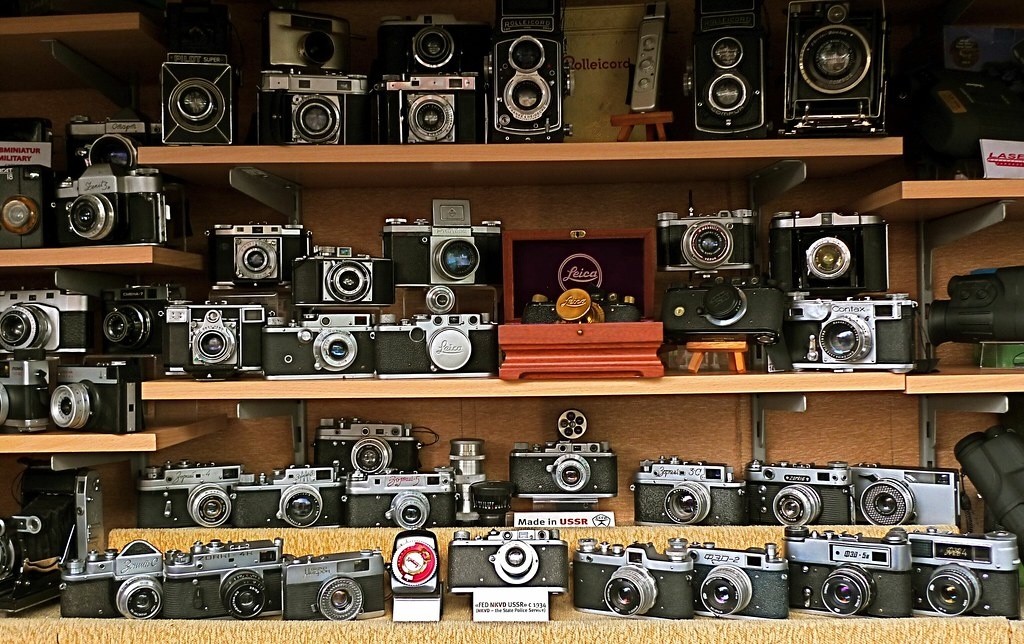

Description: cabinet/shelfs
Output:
[0,13,1024,452]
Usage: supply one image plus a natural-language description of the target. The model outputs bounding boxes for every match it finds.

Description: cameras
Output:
[0,0,1024,620]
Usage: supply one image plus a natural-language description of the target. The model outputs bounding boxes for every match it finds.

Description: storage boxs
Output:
[499,227,666,380]
